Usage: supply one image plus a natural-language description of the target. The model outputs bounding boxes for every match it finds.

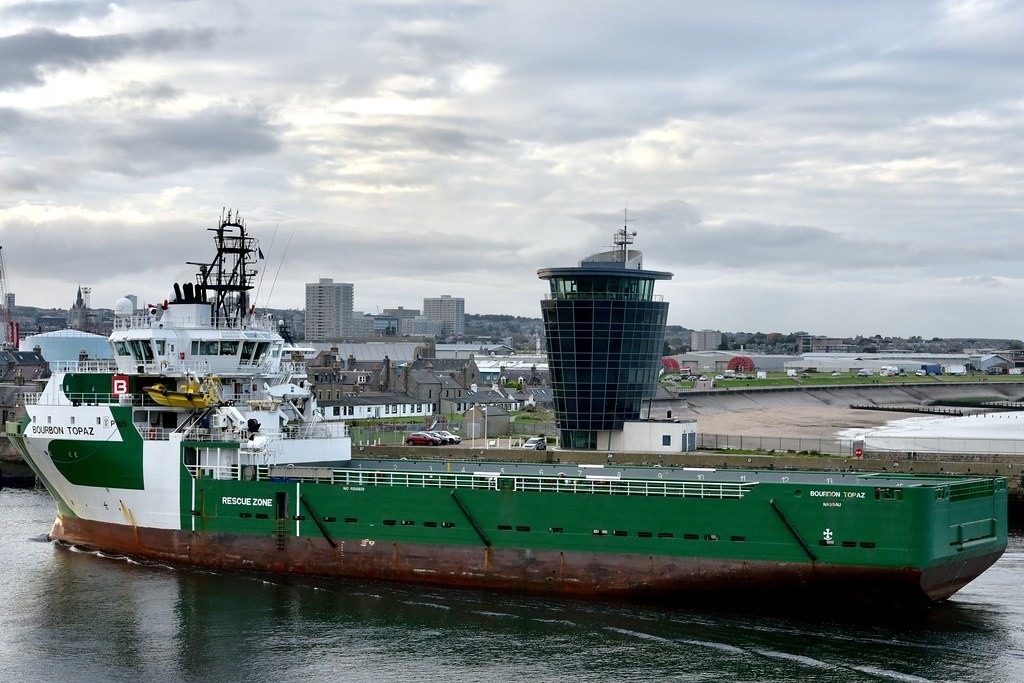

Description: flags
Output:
[259,248,264,260]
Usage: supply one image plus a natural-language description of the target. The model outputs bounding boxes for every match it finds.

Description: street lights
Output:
[469,405,474,447]
[482,405,487,447]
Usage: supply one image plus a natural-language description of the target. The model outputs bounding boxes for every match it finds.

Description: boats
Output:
[5,205,1009,612]
[143,377,221,409]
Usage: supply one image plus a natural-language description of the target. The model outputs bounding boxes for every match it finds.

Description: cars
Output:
[832,372,841,376]
[688,376,697,381]
[715,375,724,381]
[442,431,461,444]
[522,438,546,450]
[895,372,899,375]
[747,373,755,379]
[699,375,708,381]
[419,431,450,445]
[673,377,681,382]
[900,373,908,376]
[664,375,673,380]
[721,373,729,378]
[800,373,810,378]
[736,373,747,379]
[406,433,441,446]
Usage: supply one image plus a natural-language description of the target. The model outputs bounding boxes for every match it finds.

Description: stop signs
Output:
[855,449,862,456]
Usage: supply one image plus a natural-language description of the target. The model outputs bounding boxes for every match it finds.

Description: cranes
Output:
[0,245,12,349]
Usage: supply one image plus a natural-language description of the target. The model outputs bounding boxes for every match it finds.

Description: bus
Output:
[880,366,898,377]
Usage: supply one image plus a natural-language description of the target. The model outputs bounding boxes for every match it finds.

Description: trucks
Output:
[787,370,797,377]
[725,370,735,377]
[858,369,873,377]
[915,370,926,377]
[942,365,967,375]
[680,365,703,380]
